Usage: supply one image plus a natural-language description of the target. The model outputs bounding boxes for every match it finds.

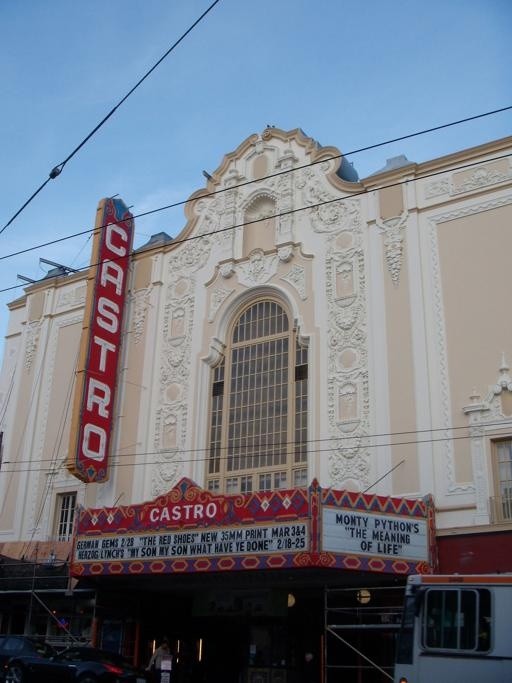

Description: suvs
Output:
[1,634,57,680]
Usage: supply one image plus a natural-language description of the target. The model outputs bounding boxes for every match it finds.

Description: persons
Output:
[145,639,170,683]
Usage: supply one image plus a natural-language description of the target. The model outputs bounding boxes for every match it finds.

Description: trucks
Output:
[393,573,511,682]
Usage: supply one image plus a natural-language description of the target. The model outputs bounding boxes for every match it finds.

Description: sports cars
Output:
[3,635,155,683]
